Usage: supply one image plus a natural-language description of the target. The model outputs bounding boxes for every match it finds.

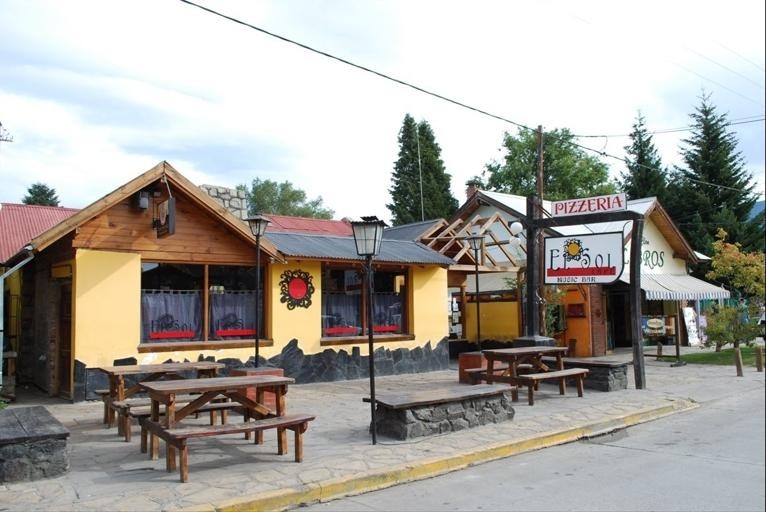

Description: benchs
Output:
[93,389,195,424]
[362,384,517,442]
[129,401,251,453]
[0,406,70,484]
[519,368,589,406]
[162,414,316,482]
[540,357,633,392]
[112,394,230,442]
[464,364,533,388]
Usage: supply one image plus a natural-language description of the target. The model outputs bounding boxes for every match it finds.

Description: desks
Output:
[482,346,568,403]
[139,374,295,473]
[99,361,226,436]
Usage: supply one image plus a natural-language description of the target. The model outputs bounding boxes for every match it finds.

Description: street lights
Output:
[350,216,388,444]
[242,212,272,367]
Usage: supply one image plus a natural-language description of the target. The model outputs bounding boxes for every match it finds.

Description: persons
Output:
[737,297,750,326]
[756,302,766,342]
